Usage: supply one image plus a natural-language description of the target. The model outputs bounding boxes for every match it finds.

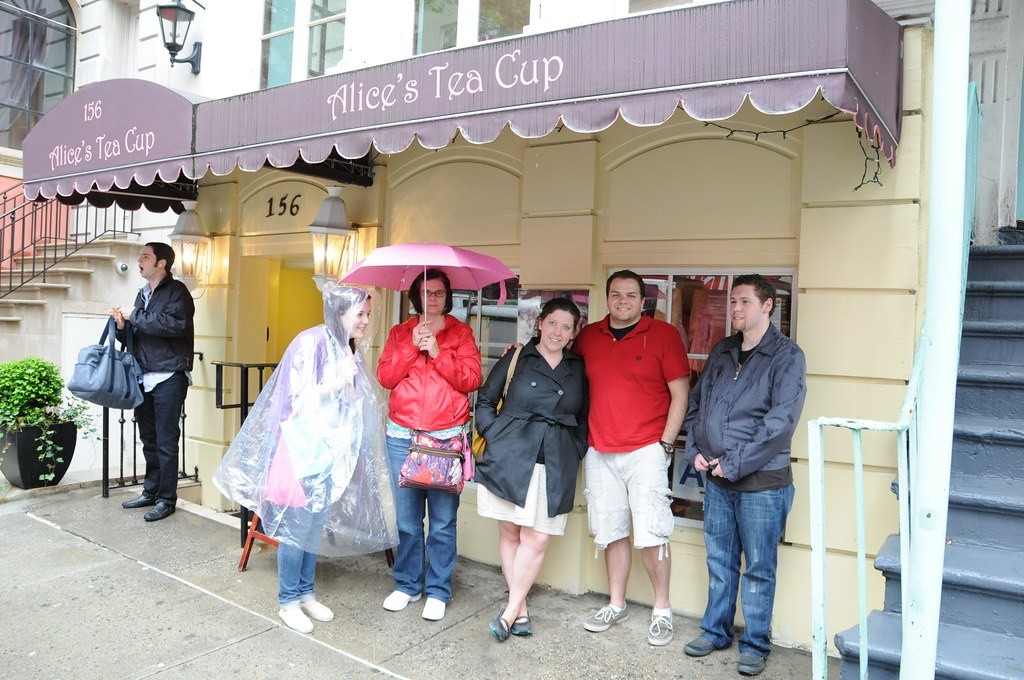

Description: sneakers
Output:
[422,597,446,621]
[647,608,674,646]
[384,590,421,611]
[301,599,334,622]
[583,602,630,632]
[279,602,314,634]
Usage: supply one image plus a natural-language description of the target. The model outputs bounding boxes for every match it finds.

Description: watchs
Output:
[659,440,675,454]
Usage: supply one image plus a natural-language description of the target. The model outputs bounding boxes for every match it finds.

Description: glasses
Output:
[420,289,447,297]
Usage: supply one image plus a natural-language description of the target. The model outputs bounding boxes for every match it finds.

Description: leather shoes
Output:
[144,502,176,520]
[122,496,155,509]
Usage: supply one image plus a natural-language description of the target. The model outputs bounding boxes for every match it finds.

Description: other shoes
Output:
[511,610,532,635]
[489,608,510,642]
[684,635,716,656]
[737,653,765,674]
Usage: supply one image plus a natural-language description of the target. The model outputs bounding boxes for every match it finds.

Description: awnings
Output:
[23,0,902,215]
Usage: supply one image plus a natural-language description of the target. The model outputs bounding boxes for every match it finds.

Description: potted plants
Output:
[0,358,93,491]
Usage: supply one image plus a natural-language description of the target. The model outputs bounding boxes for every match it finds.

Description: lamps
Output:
[169,200,212,299]
[155,0,209,75]
[308,196,361,293]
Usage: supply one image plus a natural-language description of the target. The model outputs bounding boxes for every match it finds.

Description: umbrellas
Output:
[337,243,519,326]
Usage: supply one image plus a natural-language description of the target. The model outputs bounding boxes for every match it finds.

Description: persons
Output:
[261,286,372,633]
[473,296,589,643]
[377,268,483,620]
[684,273,808,675]
[500,269,691,645]
[110,242,195,521]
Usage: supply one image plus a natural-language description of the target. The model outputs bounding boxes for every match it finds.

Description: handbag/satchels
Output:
[66,313,144,409]
[471,399,504,459]
[398,429,464,495]
